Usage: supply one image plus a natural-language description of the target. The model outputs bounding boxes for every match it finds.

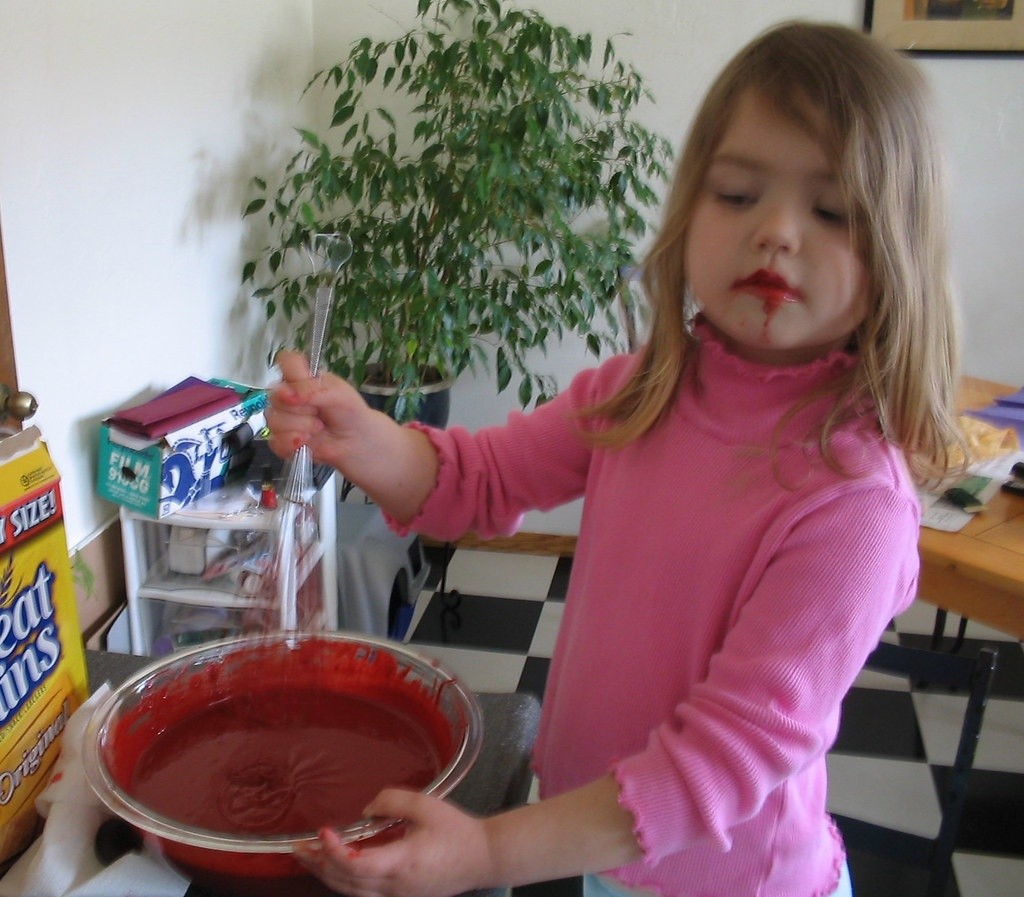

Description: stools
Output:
[336,501,430,639]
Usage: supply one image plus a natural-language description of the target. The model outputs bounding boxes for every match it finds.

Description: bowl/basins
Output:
[79,629,484,897]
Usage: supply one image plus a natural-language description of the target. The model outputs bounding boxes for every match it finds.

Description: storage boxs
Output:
[98,377,270,520]
[1,424,89,865]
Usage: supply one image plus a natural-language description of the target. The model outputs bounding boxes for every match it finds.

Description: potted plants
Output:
[242,0,676,432]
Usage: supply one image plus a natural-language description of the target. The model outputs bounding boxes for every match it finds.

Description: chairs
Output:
[826,639,999,897]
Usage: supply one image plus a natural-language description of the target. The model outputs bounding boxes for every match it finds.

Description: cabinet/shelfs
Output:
[118,470,338,657]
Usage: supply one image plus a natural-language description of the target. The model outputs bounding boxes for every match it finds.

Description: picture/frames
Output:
[865,0,1024,59]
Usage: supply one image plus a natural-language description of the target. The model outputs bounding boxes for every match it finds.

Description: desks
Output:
[85,651,542,896]
[917,376,1024,640]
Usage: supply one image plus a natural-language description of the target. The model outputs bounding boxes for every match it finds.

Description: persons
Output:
[266,19,967,897]
